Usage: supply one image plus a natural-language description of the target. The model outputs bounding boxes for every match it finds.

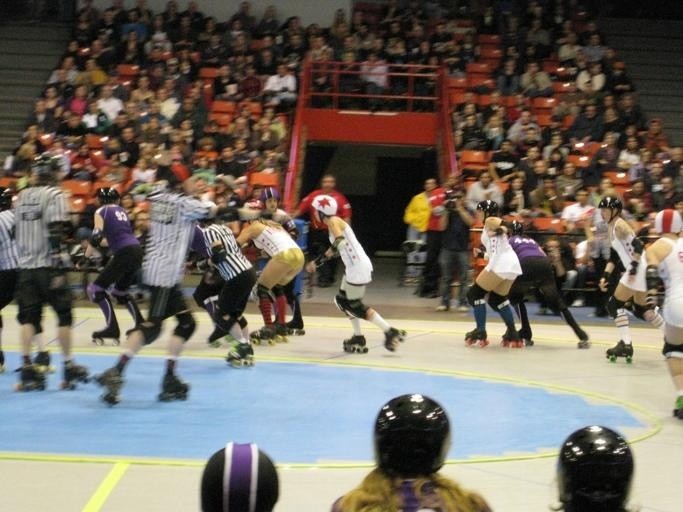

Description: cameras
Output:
[445,191,462,210]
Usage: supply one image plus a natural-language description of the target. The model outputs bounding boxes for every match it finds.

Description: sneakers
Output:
[436,299,472,312]
[517,328,534,346]
[570,299,587,307]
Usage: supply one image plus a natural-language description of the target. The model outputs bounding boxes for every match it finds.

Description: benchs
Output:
[0,7,300,222]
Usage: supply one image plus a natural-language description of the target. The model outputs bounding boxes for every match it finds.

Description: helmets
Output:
[312,194,339,217]
[97,186,120,202]
[556,424,634,504]
[202,201,218,219]
[165,160,193,188]
[507,220,523,236]
[476,199,499,217]
[243,198,266,211]
[598,195,624,211]
[0,184,12,210]
[374,392,451,478]
[32,153,60,173]
[260,185,280,202]
[654,207,683,235]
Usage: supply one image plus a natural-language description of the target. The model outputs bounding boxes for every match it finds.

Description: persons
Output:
[546,421,637,511]
[11,153,91,395]
[636,205,683,416]
[91,164,217,395]
[327,391,492,511]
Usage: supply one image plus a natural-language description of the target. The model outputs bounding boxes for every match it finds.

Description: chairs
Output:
[406,0,683,293]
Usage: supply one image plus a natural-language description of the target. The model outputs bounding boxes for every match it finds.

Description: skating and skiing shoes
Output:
[95,365,123,406]
[60,365,92,390]
[158,372,189,402]
[383,327,407,352]
[465,327,490,348]
[576,330,592,350]
[672,395,683,422]
[501,328,524,349]
[342,334,370,354]
[91,325,138,348]
[207,324,234,348]
[14,351,57,393]
[225,342,255,369]
[605,339,634,363]
[248,319,306,346]
